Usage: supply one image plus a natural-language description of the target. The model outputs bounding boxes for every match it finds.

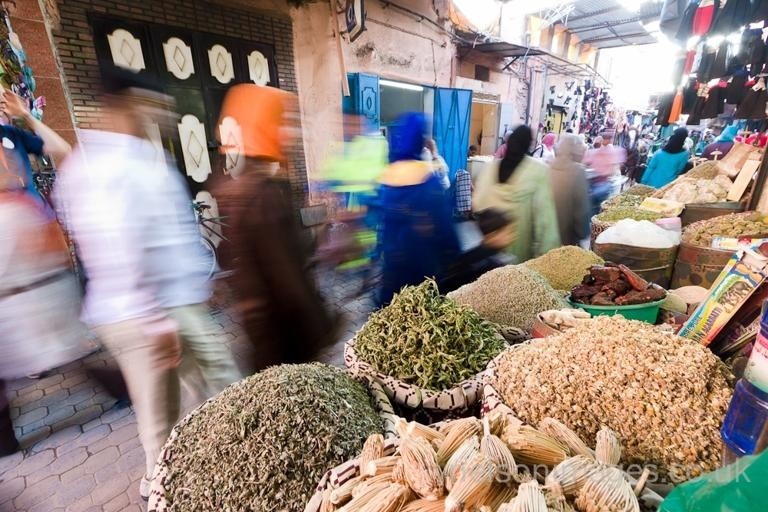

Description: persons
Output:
[0,83,105,380]
[323,108,520,316]
[473,105,738,274]
[198,86,352,371]
[54,64,242,480]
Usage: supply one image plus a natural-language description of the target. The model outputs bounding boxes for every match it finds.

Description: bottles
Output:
[655,218,682,240]
[720,299,768,459]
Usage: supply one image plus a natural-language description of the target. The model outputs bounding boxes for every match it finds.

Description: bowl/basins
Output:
[565,286,668,326]
